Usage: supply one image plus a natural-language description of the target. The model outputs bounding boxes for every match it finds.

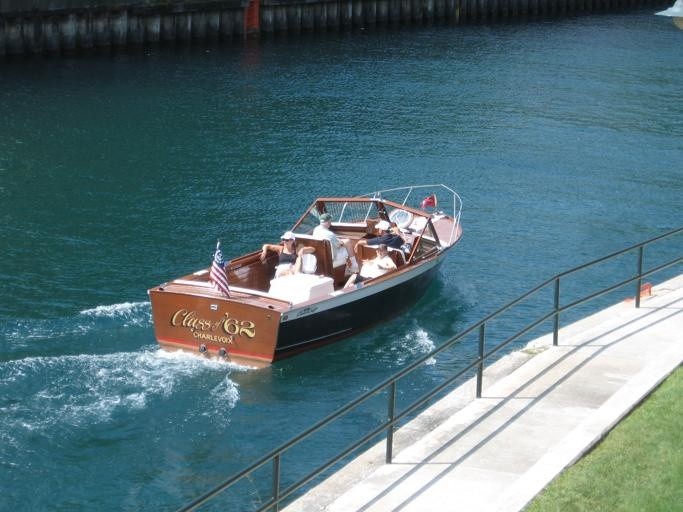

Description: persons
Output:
[313,214,349,262]
[261,232,316,278]
[354,221,406,253]
[344,244,397,288]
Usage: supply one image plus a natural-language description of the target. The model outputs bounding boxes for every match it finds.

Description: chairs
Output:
[294,234,406,288]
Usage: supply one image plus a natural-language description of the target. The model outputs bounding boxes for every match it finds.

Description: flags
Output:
[420,194,435,208]
[209,242,231,299]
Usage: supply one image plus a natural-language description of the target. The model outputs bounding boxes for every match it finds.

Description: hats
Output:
[377,244,386,250]
[280,231,296,239]
[319,213,332,221]
[375,220,390,231]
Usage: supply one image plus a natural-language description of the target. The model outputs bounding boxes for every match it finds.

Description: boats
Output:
[146,183,462,362]
